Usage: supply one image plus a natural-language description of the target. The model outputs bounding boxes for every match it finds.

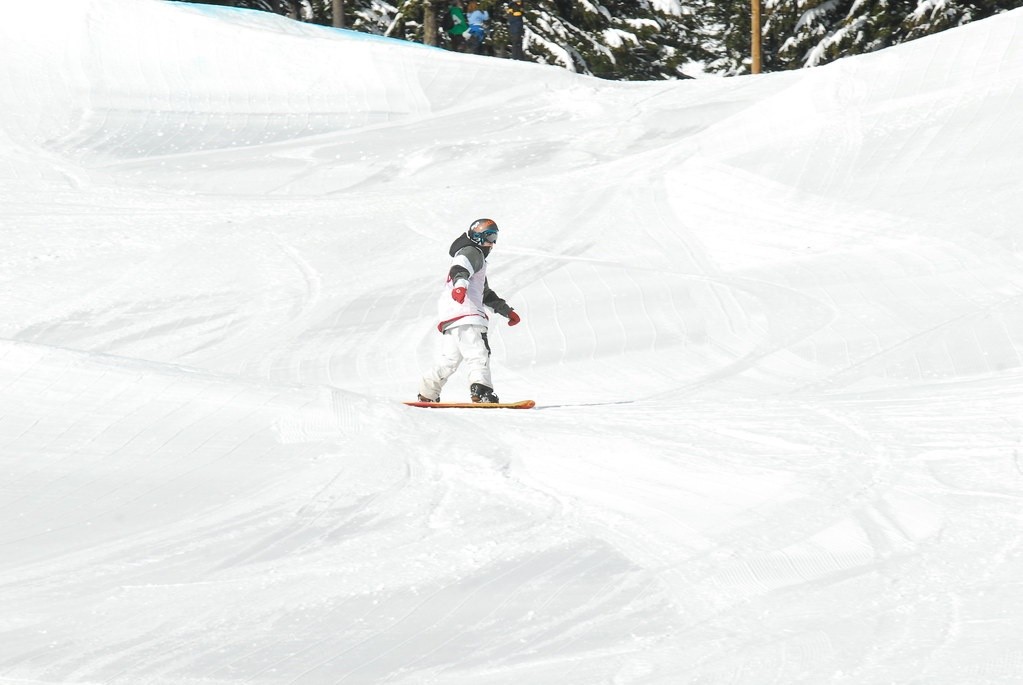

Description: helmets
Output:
[468,219,499,245]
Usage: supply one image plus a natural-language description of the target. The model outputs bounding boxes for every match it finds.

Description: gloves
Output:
[452,287,467,304]
[507,310,520,326]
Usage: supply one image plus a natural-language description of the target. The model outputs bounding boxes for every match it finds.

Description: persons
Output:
[506,0,525,60]
[466,0,489,54]
[448,0,468,52]
[418,219,521,404]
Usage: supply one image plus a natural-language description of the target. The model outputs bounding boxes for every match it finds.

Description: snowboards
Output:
[404,400,536,409]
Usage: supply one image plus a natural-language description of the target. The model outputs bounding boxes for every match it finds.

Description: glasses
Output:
[484,230,497,243]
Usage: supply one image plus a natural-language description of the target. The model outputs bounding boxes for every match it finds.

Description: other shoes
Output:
[418,393,440,402]
[471,383,499,403]
